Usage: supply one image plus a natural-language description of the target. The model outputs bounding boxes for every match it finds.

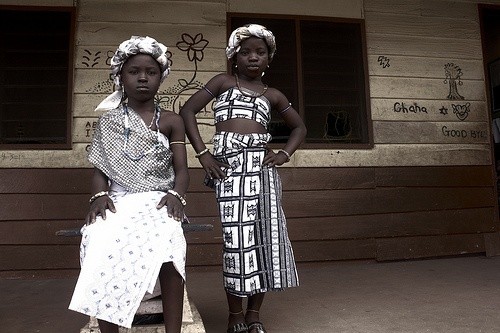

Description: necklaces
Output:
[123,101,161,161]
[235,72,268,98]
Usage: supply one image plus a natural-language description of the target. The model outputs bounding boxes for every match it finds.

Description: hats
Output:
[225,23,277,60]
[111,35,171,101]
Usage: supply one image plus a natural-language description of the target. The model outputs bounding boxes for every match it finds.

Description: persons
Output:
[180,24,308,333]
[83,35,189,333]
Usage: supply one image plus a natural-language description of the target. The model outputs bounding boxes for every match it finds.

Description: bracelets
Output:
[167,189,187,206]
[277,149,291,163]
[89,191,109,206]
[195,148,209,158]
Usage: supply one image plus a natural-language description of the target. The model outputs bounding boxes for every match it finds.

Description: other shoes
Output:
[227,322,268,333]
